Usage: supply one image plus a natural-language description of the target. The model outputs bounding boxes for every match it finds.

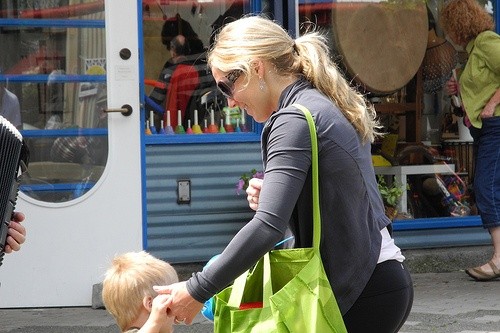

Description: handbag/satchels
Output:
[212,103,348,333]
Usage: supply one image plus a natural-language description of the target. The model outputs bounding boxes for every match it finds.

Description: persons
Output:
[153,12,414,333]
[160,34,202,130]
[102,249,180,333]
[144,12,219,130]
[4,212,26,254]
[437,0,500,281]
[0,37,111,203]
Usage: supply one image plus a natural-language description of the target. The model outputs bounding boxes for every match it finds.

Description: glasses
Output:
[217,66,243,97]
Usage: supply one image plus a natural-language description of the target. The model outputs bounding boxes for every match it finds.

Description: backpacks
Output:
[181,65,226,133]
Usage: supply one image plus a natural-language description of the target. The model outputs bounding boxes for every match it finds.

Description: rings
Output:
[174,317,186,324]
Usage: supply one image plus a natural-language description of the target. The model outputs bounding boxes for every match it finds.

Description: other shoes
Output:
[464,261,500,282]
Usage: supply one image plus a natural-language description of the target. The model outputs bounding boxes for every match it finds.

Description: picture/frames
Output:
[37,56,66,114]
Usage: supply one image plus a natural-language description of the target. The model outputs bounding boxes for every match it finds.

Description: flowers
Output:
[235,170,264,194]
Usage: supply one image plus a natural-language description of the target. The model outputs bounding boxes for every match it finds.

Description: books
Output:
[448,62,464,108]
